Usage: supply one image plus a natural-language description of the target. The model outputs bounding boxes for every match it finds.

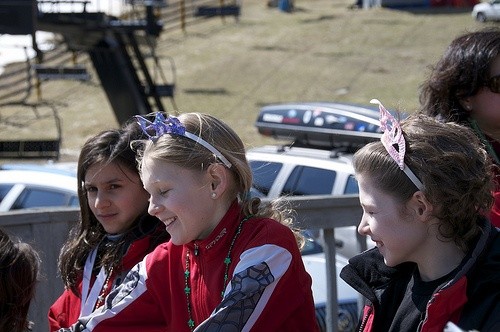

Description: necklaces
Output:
[183,214,251,330]
[96,263,112,310]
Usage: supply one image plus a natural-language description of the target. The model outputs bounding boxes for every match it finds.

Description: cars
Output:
[249,177,378,332]
[472,0,500,23]
[0,170,93,212]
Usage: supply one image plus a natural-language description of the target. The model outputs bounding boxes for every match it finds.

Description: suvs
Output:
[244,101,414,264]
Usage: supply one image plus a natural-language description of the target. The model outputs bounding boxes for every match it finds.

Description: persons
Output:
[339,98,500,332]
[416,26,500,229]
[59,112,314,332]
[48,128,170,332]
[0,229,46,332]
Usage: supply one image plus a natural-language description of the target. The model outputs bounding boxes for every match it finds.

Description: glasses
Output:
[482,75,500,93]
